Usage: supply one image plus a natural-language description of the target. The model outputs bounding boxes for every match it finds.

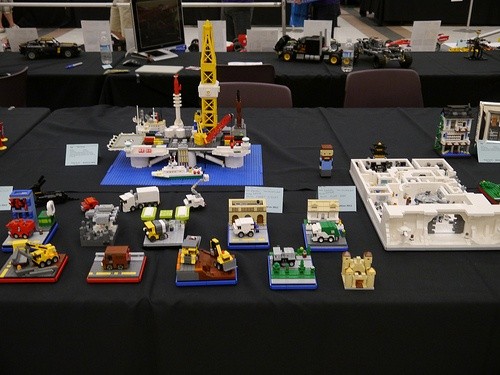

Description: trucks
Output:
[312,221,339,243]
[6,219,35,239]
[143,219,169,241]
[272,247,295,268]
[233,217,254,237]
[102,246,130,270]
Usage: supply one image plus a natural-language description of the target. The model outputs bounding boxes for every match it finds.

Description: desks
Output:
[0,0,500,374]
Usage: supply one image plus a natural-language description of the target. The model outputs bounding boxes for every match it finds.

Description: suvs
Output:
[19,37,82,60]
[275,30,359,64]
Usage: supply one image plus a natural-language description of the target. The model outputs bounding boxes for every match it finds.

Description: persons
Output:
[0,0,22,33]
[109,0,136,40]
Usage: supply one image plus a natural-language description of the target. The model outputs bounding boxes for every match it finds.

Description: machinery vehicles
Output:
[181,235,201,265]
[12,239,60,268]
[210,238,239,272]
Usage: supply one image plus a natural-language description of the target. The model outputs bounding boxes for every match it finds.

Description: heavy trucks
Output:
[118,186,161,212]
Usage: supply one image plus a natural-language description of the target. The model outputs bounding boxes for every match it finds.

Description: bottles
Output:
[100,32,112,64]
[342,38,354,72]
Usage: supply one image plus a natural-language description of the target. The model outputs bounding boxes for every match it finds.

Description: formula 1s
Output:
[354,36,412,68]
